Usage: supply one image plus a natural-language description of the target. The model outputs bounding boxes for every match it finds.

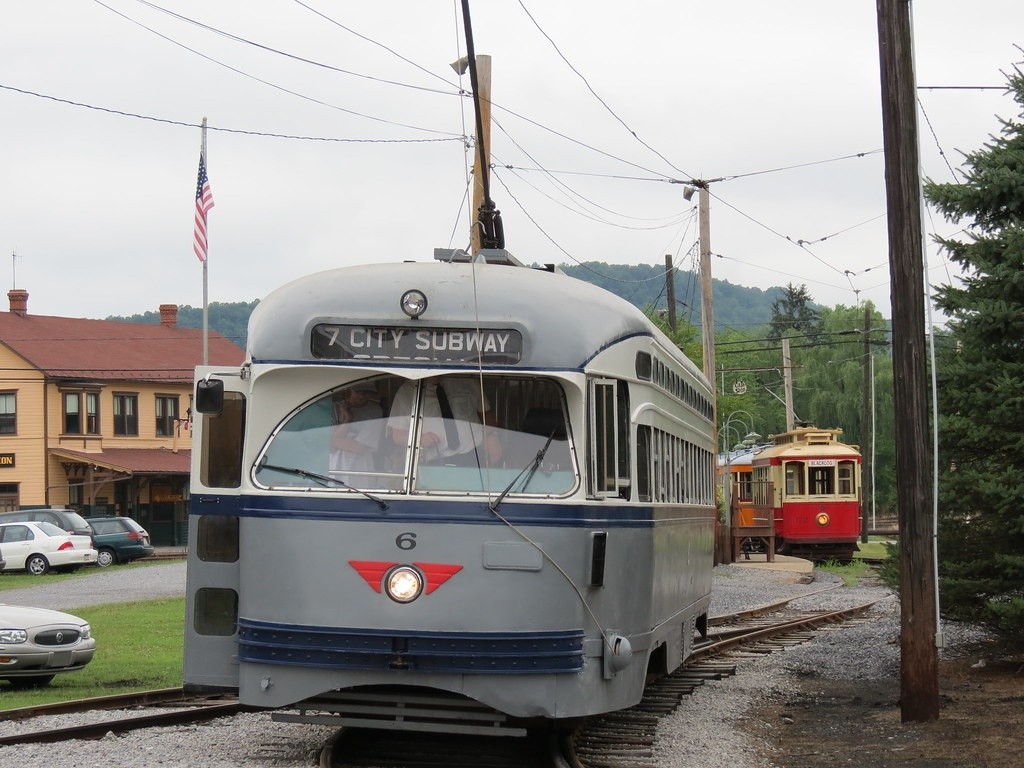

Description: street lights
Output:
[726,410,763,538]
[717,419,755,500]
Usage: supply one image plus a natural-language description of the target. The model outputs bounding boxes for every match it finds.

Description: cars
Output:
[0,603,96,686]
[0,521,99,577]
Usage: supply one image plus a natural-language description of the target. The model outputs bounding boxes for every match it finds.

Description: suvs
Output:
[86,516,155,567]
[0,509,96,573]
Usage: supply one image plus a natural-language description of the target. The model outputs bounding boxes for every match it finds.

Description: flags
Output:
[192,153,216,263]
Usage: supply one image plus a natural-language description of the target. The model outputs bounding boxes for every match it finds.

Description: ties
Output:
[435,383,460,450]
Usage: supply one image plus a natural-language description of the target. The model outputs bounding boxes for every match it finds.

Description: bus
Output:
[181,257,716,737]
[717,427,863,565]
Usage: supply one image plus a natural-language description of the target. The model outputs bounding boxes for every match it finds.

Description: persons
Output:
[330,389,383,481]
[385,378,501,468]
